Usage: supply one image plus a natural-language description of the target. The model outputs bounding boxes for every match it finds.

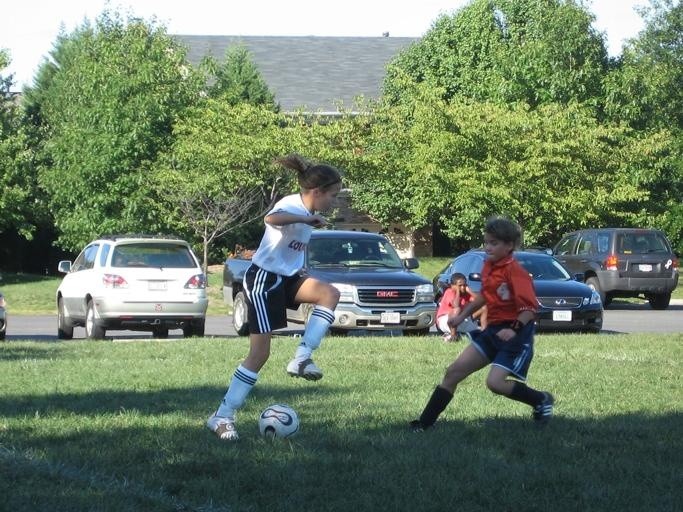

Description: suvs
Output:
[55,232,209,341]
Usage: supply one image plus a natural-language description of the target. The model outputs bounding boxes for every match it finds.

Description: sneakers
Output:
[206,411,239,440]
[442,333,461,342]
[533,393,554,428]
[286,358,323,380]
[409,421,436,432]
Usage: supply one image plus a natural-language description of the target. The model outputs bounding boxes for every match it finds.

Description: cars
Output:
[0,275,8,339]
[431,247,608,335]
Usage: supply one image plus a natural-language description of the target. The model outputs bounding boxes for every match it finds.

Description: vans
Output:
[542,225,680,310]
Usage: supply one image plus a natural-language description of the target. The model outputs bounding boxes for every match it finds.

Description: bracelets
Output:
[506,320,523,335]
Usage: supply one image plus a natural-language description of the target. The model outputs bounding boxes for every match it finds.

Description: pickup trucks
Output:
[221,228,438,336]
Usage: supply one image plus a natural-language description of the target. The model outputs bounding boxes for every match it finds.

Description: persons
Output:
[203,149,343,443]
[434,272,487,343]
[401,213,554,436]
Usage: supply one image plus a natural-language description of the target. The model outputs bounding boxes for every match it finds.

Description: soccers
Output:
[258,403,298,438]
